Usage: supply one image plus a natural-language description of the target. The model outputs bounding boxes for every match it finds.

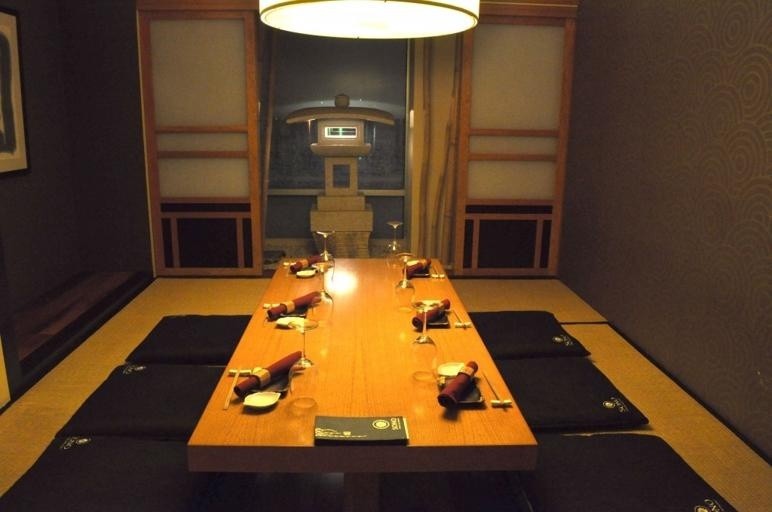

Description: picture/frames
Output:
[0,12,31,176]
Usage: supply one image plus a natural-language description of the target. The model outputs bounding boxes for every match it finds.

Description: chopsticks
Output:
[451,309,466,328]
[222,363,241,410]
[480,372,505,406]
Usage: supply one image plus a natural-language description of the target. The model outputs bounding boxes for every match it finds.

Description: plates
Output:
[277,315,301,326]
[245,390,281,409]
[440,360,464,379]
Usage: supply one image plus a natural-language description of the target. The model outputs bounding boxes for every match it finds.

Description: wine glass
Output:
[288,227,335,408]
[386,221,438,383]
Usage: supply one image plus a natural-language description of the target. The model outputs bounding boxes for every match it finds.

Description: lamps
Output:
[259,0,482,42]
